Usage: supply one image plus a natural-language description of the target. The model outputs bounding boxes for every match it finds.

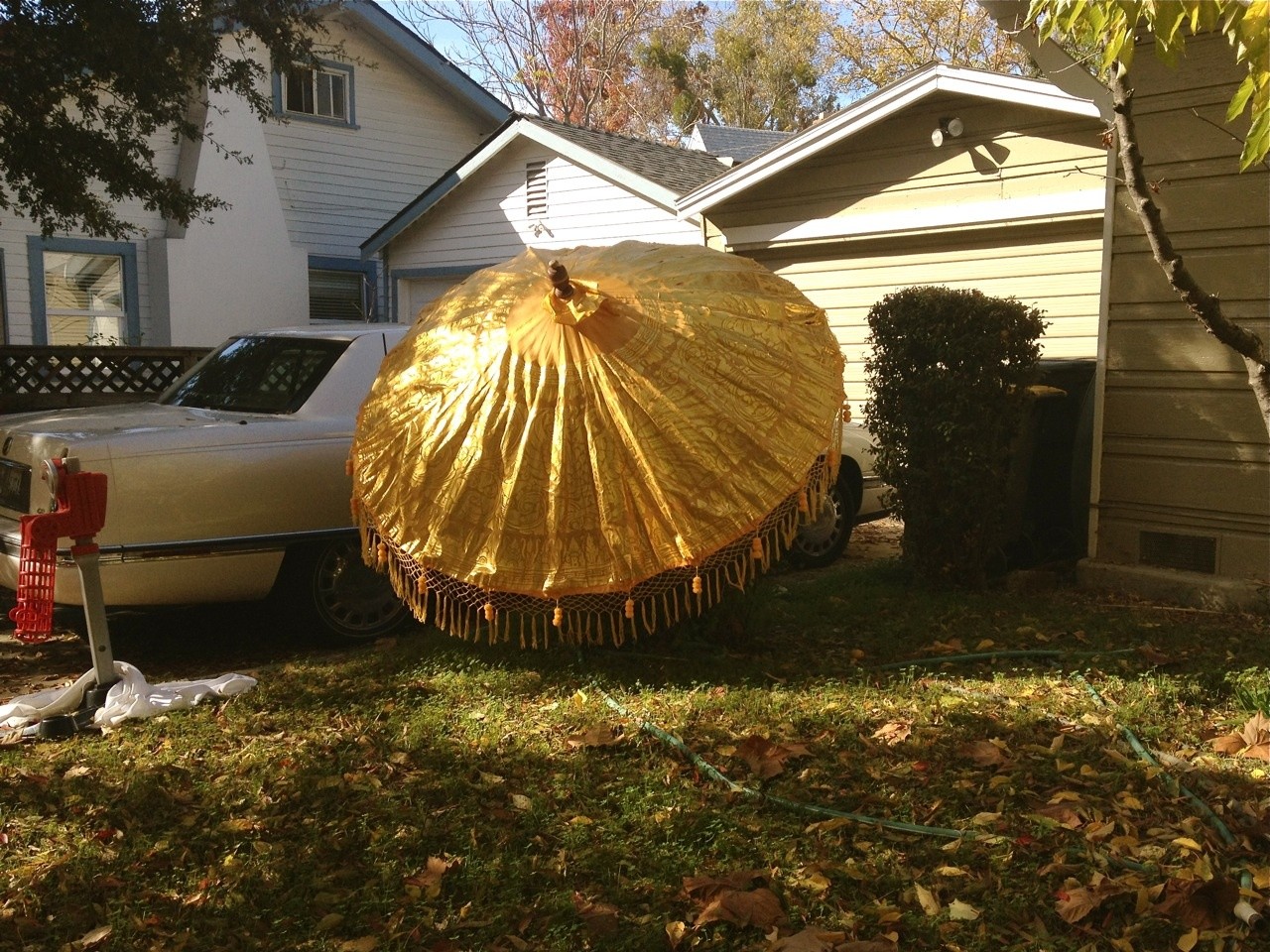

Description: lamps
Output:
[931,116,964,147]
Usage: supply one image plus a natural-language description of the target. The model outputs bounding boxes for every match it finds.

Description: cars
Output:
[2,322,889,642]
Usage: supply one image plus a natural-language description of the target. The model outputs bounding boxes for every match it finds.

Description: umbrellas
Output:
[346,240,851,648]
